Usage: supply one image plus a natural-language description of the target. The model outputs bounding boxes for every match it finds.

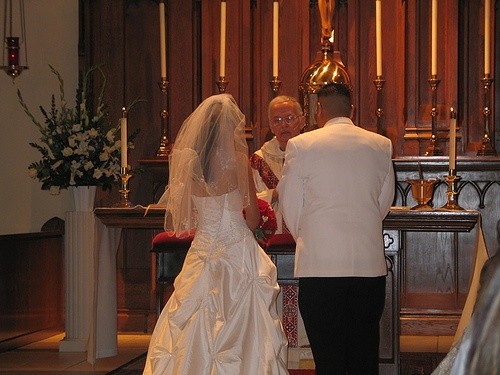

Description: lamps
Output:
[0,0,29,84]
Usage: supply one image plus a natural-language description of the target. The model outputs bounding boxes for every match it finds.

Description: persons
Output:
[274,82,396,375]
[141,93,291,375]
[250,96,315,369]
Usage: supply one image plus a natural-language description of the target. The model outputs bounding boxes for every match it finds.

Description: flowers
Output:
[243,197,278,249]
[17,64,147,195]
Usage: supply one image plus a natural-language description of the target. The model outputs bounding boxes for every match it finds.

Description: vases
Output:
[71,185,96,211]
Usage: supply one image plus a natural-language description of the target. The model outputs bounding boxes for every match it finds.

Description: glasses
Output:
[269,112,304,127]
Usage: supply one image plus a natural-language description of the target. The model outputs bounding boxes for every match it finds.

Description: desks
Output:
[150,212,480,375]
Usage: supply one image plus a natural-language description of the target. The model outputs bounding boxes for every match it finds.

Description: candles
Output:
[449,113,456,175]
[273,2,278,79]
[159,2,166,79]
[121,112,127,175]
[375,0,382,77]
[484,0,490,75]
[432,0,437,75]
[220,1,226,78]
